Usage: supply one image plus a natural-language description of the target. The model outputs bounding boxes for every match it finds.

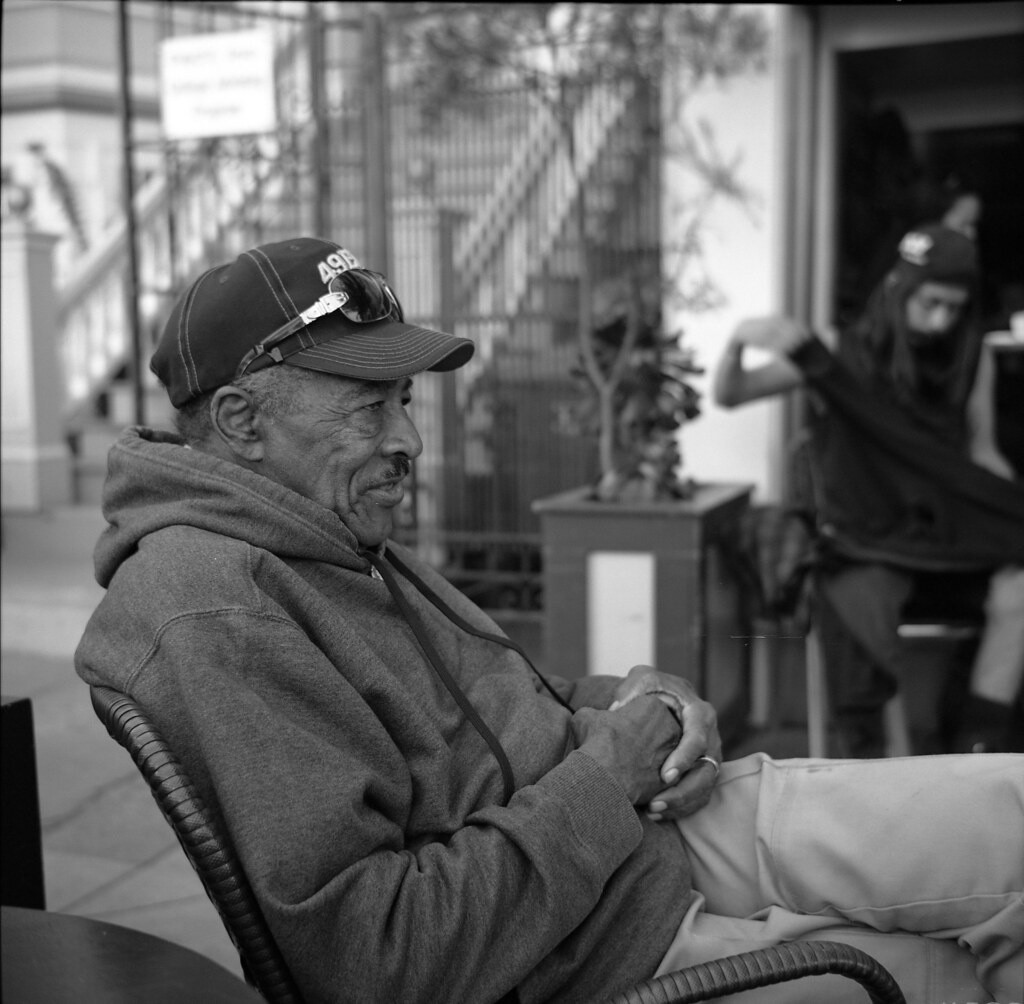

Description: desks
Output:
[0,904,270,1004]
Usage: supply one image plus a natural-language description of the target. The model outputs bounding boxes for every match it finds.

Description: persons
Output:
[714,227,1024,759]
[74,237,1024,1004]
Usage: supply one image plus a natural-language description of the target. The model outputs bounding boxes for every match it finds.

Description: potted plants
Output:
[398,1,777,755]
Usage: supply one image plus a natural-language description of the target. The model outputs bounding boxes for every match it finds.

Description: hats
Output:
[897,225,980,287]
[150,237,474,409]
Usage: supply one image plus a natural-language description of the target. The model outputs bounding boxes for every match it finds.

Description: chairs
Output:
[88,681,912,1003]
[749,314,1024,757]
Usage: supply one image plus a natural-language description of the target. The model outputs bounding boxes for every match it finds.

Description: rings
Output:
[697,755,721,777]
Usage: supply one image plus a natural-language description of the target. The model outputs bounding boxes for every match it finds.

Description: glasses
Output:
[234,268,404,387]
